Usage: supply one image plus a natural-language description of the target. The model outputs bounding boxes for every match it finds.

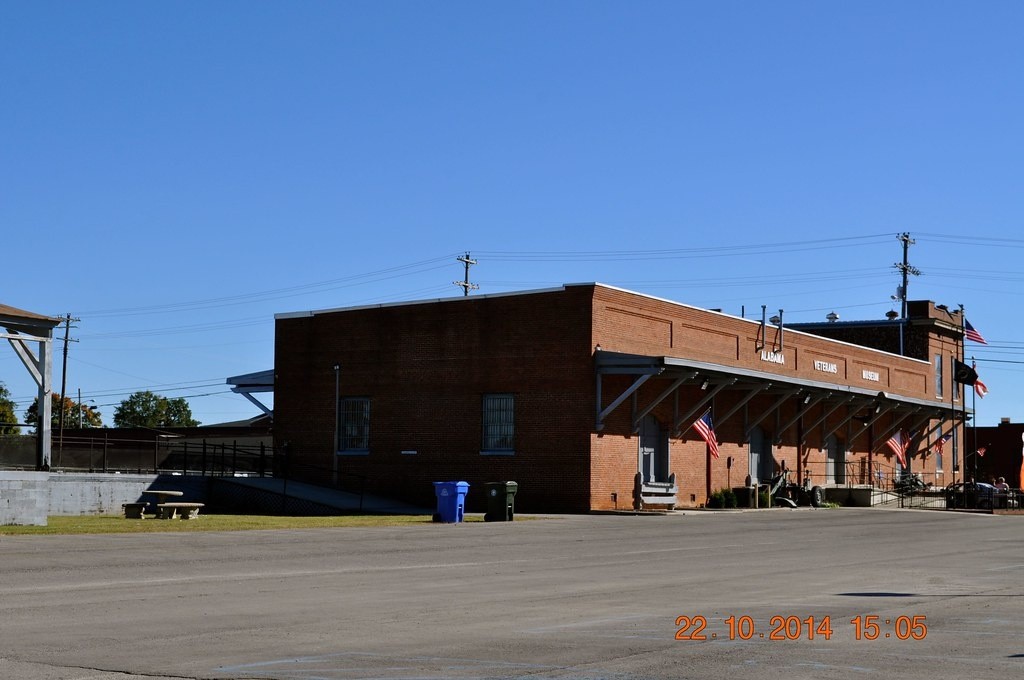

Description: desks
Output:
[142,486,184,520]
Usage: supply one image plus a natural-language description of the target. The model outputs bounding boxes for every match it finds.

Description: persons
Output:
[995,477,1009,491]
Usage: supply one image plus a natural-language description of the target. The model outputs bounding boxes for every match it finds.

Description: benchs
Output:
[122,501,205,520]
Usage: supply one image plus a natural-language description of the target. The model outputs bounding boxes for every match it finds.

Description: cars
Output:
[945,482,1018,509]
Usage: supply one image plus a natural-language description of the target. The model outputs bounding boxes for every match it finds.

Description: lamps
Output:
[875,403,882,414]
[700,378,709,390]
[803,392,811,406]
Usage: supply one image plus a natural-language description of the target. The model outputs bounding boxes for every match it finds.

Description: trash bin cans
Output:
[484,481,518,522]
[432,480,470,523]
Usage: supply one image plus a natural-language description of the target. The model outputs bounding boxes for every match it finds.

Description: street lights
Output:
[67,399,95,428]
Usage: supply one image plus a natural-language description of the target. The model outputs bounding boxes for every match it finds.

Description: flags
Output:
[886,429,909,469]
[976,445,988,456]
[964,318,988,344]
[973,363,989,399]
[935,435,952,455]
[693,410,719,460]
